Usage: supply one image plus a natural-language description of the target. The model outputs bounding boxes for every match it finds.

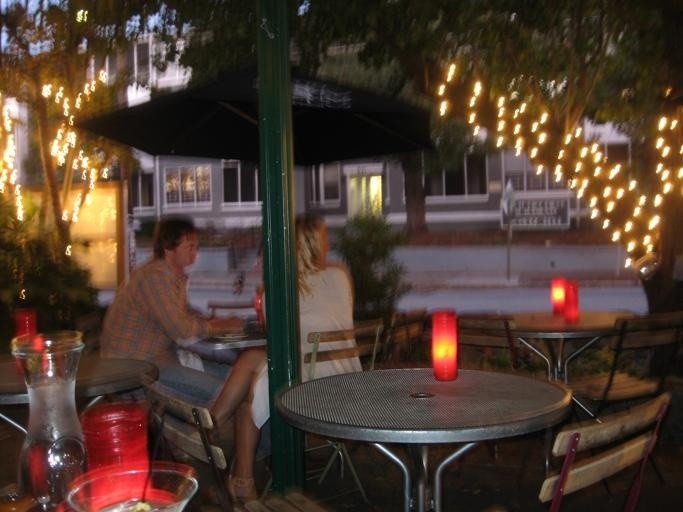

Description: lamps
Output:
[15,309,39,369]
[549,277,569,315]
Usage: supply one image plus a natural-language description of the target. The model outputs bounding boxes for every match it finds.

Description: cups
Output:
[80,401,150,493]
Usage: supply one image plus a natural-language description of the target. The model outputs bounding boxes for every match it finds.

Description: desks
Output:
[0,357,158,439]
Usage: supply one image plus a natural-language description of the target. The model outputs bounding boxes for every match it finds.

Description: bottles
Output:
[10,328,92,512]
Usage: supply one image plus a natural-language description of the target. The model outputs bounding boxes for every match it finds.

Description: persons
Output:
[180,212,364,512]
[94,214,232,401]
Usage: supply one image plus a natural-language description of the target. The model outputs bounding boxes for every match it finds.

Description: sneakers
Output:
[206,476,259,505]
[174,432,204,460]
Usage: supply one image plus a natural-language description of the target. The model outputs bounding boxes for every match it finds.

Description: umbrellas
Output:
[76,70,441,168]
